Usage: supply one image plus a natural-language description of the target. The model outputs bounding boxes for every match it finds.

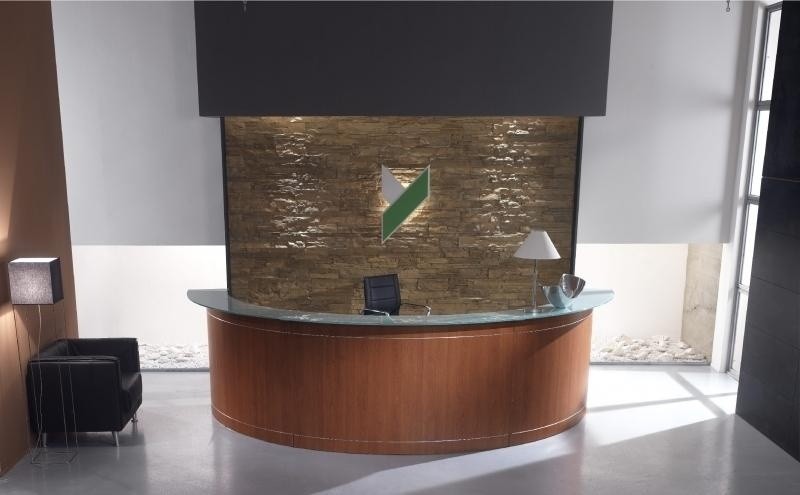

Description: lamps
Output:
[512,229,561,314]
[8,257,81,466]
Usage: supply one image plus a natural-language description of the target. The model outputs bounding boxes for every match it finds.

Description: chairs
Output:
[29,335,144,450]
[360,272,431,317]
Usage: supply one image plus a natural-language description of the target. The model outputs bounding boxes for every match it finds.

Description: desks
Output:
[186,288,615,455]
